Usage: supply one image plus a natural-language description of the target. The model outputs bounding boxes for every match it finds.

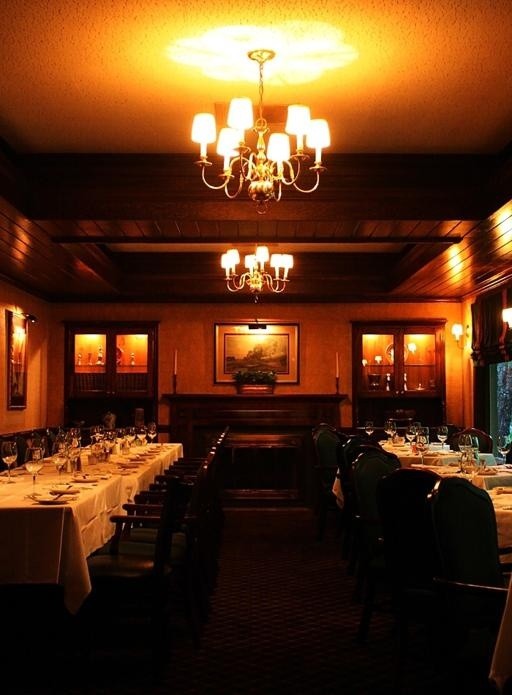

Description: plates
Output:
[385,343,412,364]
[36,494,73,504]
[48,484,84,494]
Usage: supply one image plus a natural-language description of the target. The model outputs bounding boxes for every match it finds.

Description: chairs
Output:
[310,421,511,693]
[0,418,235,695]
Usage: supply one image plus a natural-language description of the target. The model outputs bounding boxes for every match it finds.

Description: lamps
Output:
[220,245,297,304]
[451,324,470,351]
[24,313,38,323]
[187,46,333,217]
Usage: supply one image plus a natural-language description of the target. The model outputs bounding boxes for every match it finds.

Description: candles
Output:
[173,348,178,375]
[335,352,339,378]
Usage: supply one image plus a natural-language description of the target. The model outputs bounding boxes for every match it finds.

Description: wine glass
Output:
[2,442,18,484]
[49,423,160,482]
[363,416,509,477]
[24,447,45,497]
[375,355,383,365]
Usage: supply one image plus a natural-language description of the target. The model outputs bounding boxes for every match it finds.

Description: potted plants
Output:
[230,368,277,395]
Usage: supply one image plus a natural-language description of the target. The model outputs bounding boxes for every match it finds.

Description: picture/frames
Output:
[6,309,29,409]
[212,322,300,385]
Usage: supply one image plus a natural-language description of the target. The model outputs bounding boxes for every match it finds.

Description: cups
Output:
[368,373,381,390]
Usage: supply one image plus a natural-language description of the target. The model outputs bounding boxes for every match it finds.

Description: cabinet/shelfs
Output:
[350,315,448,427]
[59,316,162,428]
